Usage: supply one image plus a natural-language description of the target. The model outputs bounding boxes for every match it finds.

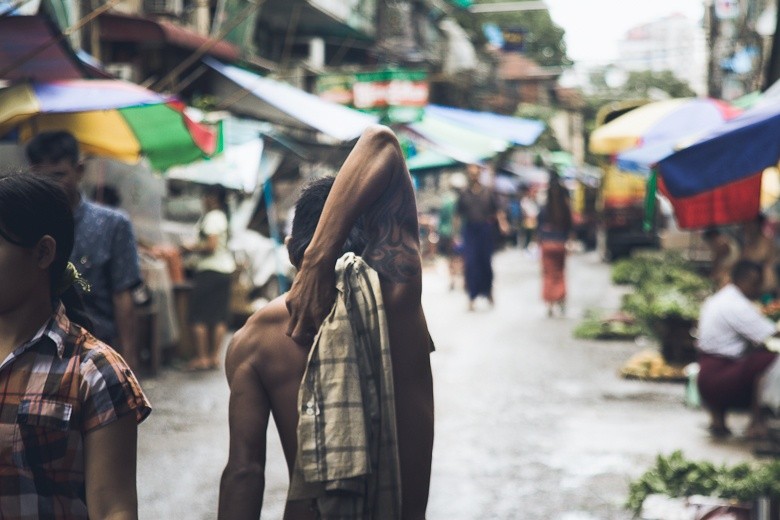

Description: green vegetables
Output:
[574,256,710,338]
[627,449,780,520]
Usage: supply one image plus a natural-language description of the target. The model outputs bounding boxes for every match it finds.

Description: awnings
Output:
[97,14,241,59]
[616,75,780,198]
[204,58,377,141]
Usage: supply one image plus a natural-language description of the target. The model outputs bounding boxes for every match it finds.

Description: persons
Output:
[25,128,145,382]
[218,122,436,520]
[177,185,237,371]
[520,169,574,318]
[431,170,464,290]
[452,164,510,312]
[703,211,780,295]
[0,175,153,520]
[90,183,120,209]
[696,259,780,441]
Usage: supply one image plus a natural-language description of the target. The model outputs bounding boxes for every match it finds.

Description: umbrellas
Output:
[589,95,747,155]
[0,77,225,174]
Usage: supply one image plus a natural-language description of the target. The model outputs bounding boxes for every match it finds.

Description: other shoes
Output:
[183,358,211,372]
[709,423,731,438]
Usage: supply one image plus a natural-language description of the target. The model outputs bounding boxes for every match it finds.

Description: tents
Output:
[0,0,121,82]
